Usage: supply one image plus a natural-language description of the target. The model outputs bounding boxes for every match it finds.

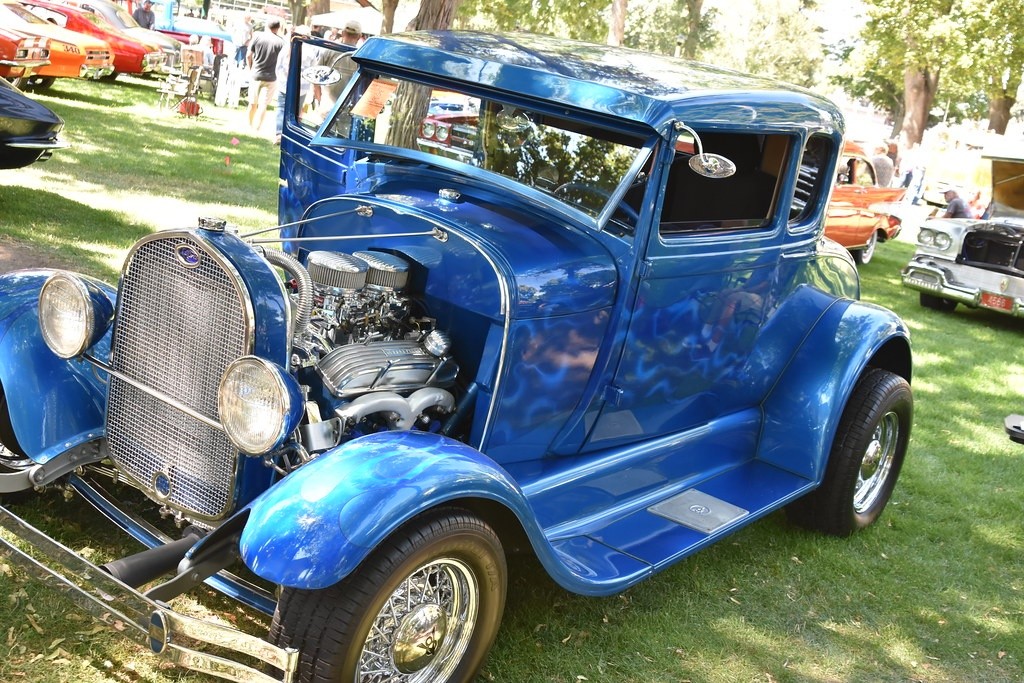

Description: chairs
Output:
[157,54,228,118]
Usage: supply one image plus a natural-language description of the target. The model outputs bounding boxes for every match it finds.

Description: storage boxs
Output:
[179,101,201,116]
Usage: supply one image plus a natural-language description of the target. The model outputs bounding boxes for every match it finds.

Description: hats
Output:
[939,185,956,193]
[344,20,362,34]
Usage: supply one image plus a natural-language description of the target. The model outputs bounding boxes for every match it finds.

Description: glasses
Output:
[147,1,155,5]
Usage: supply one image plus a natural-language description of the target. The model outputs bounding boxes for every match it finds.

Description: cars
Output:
[674,131,907,265]
[0,27,917,683]
[0,0,191,172]
[900,154,1023,323]
[353,75,541,160]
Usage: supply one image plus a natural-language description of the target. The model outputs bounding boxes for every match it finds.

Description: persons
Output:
[132,0,156,31]
[860,140,977,220]
[233,12,367,145]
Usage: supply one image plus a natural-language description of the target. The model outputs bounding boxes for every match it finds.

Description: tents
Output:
[312,8,405,35]
